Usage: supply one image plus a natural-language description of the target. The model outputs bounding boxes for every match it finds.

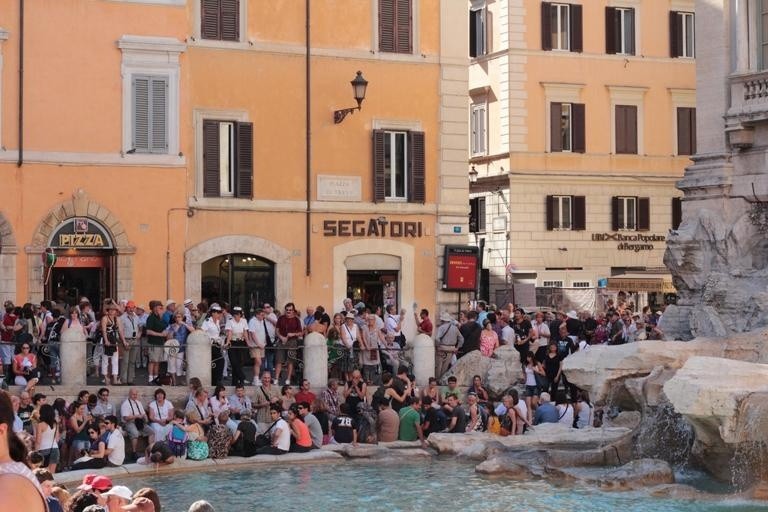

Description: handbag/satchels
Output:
[401,330,405,346]
[136,418,143,429]
[39,453,50,468]
[255,433,267,448]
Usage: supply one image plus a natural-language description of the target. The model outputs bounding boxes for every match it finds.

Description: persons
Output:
[33,468,161,512]
[188,500,215,512]
[2,296,673,472]
[1,390,49,512]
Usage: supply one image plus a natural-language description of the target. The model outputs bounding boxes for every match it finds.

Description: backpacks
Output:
[164,422,187,457]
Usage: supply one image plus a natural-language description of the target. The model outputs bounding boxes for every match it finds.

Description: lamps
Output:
[333,71,368,125]
[469,165,478,184]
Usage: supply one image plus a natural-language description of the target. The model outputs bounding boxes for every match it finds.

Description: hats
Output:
[183,299,194,306]
[125,300,137,309]
[439,311,451,322]
[100,485,133,500]
[166,299,176,305]
[345,312,355,319]
[209,306,225,313]
[232,307,242,314]
[565,310,579,319]
[86,475,113,493]
[106,304,118,312]
[135,304,145,311]
[120,496,157,511]
[76,472,97,491]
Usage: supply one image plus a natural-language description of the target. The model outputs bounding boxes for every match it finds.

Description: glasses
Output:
[298,407,303,410]
[286,309,292,311]
[104,422,109,425]
[88,432,94,435]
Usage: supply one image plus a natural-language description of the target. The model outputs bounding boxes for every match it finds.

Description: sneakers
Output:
[90,372,292,386]
